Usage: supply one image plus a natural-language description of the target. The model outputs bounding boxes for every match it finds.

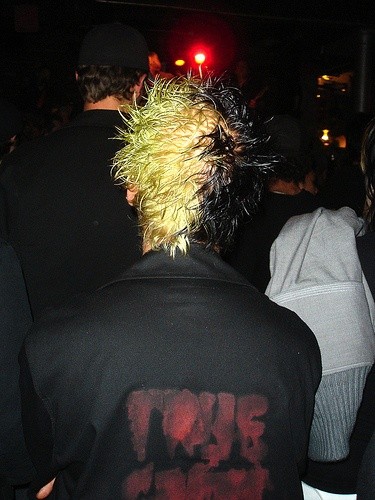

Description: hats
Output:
[78,23,151,71]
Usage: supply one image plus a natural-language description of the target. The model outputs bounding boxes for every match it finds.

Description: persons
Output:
[0,168,54,499]
[18,63,321,500]
[0,24,151,323]
[221,113,375,500]
